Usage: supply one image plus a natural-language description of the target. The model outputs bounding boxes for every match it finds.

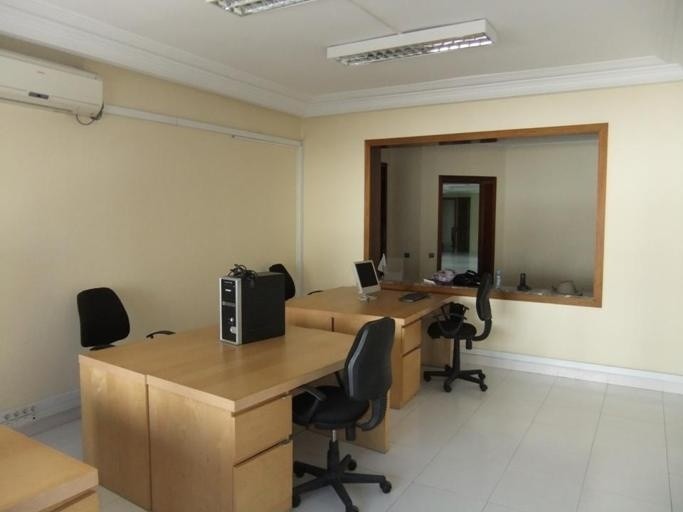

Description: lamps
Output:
[203,0,310,18]
[326,17,499,69]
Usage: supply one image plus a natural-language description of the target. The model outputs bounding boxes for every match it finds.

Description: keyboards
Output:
[398,290,427,303]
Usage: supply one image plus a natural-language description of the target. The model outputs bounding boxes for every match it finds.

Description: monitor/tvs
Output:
[351,259,381,302]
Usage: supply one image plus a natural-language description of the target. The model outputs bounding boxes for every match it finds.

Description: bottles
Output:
[517,272,530,290]
[495,271,501,288]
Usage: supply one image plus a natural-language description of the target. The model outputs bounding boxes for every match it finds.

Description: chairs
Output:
[269,263,322,300]
[423,270,495,393]
[291,316,394,508]
[78,287,174,353]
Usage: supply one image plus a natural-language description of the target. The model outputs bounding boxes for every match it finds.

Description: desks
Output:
[0,416,101,512]
[77,320,358,512]
[285,286,458,412]
[145,329,390,490]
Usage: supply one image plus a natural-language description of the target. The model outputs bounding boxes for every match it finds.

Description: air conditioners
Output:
[1,48,106,126]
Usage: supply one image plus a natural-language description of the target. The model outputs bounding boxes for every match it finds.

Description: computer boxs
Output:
[218,272,287,346]
